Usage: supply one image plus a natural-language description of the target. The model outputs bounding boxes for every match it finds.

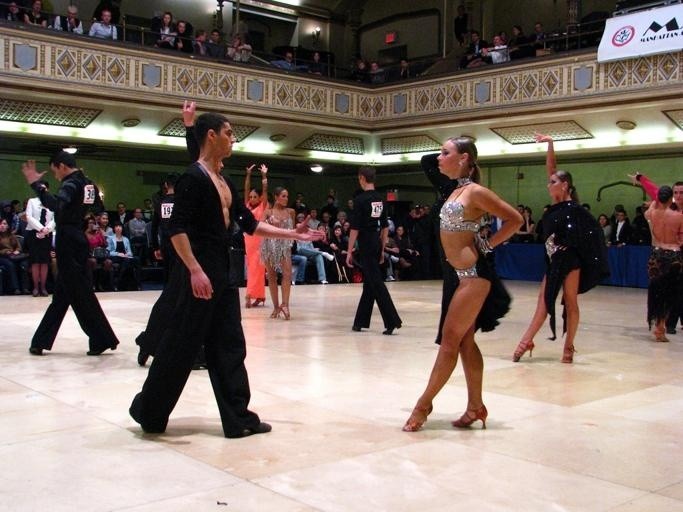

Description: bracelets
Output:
[479,239,494,255]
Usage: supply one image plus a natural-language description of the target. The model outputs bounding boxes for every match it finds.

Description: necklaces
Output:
[454,176,472,188]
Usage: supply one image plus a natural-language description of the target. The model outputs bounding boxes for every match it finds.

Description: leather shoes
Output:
[28,297,270,438]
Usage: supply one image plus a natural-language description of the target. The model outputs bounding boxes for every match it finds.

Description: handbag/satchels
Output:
[90,246,110,259]
[11,252,28,260]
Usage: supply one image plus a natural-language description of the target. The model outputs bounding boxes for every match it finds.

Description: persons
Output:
[134,98,313,370]
[1,0,328,81]
[512,131,606,364]
[582,200,636,249]
[478,202,553,244]
[82,173,179,292]
[21,151,119,356]
[290,188,360,286]
[260,186,296,321]
[128,112,271,439]
[243,163,272,309]
[401,136,525,433]
[378,202,431,282]
[346,165,403,335]
[0,180,57,297]
[626,170,683,342]
[347,22,549,83]
